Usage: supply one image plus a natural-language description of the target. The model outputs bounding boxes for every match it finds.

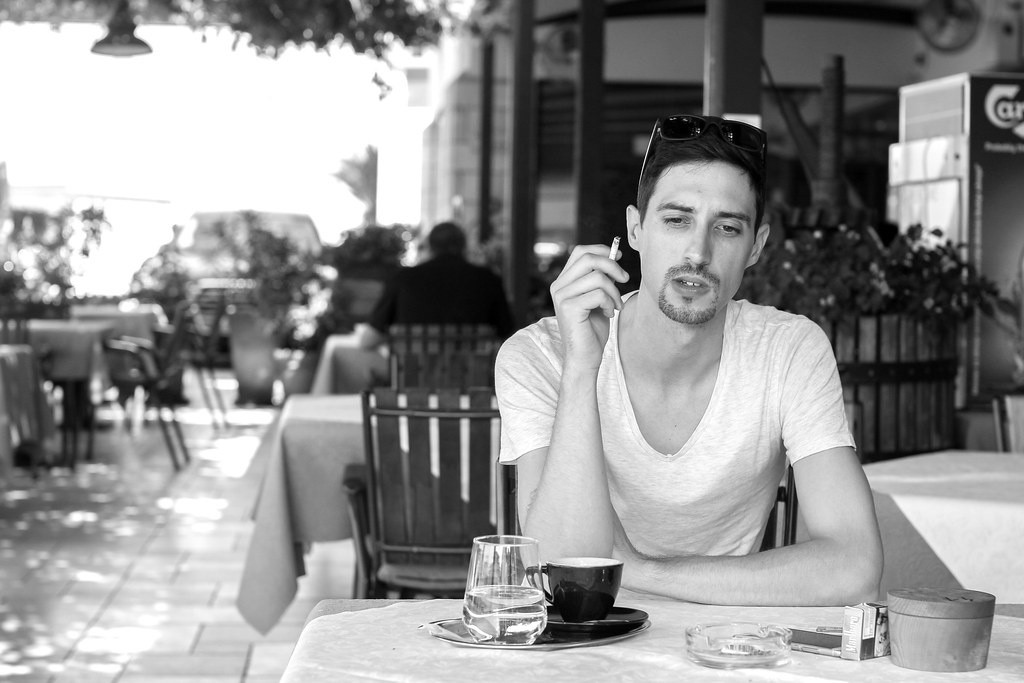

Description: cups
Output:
[463,534,546,645]
[525,557,623,624]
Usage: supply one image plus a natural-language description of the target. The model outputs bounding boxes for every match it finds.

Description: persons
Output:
[302,230,592,325]
[493,114,884,605]
[362,220,508,385]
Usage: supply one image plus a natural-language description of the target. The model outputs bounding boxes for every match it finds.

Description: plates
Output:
[548,606,648,634]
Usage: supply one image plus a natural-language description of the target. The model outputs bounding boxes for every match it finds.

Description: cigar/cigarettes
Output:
[608,236,620,260]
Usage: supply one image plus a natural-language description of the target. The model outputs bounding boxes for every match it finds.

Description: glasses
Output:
[637,114,768,187]
